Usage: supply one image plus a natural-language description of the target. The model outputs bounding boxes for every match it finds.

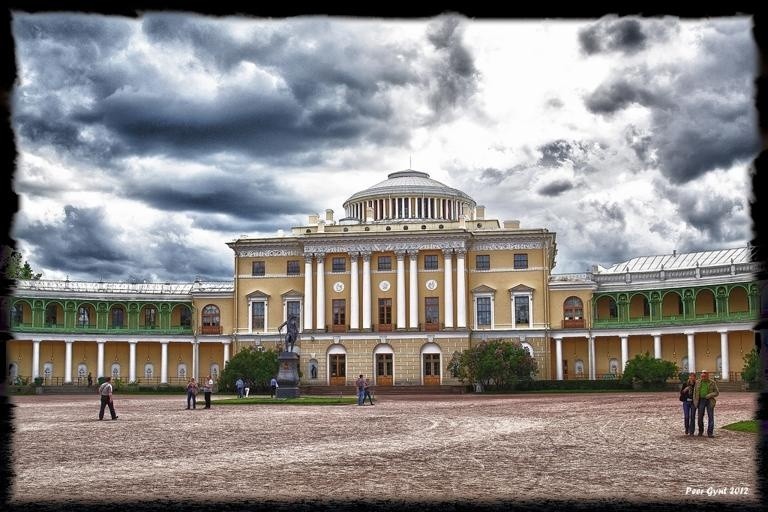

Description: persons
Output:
[235,376,244,399]
[276,310,300,353]
[363,378,375,406]
[97,377,118,420]
[201,375,213,409]
[185,377,199,410]
[692,369,720,438]
[86,371,93,388]
[679,371,697,438]
[243,378,251,398]
[269,376,279,399]
[356,374,366,406]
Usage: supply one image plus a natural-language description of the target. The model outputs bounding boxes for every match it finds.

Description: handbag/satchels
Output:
[192,386,200,396]
[679,394,687,402]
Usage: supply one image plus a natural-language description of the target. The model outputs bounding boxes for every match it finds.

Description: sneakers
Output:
[99,416,118,420]
[685,427,714,438]
[185,406,210,410]
[359,403,375,406]
